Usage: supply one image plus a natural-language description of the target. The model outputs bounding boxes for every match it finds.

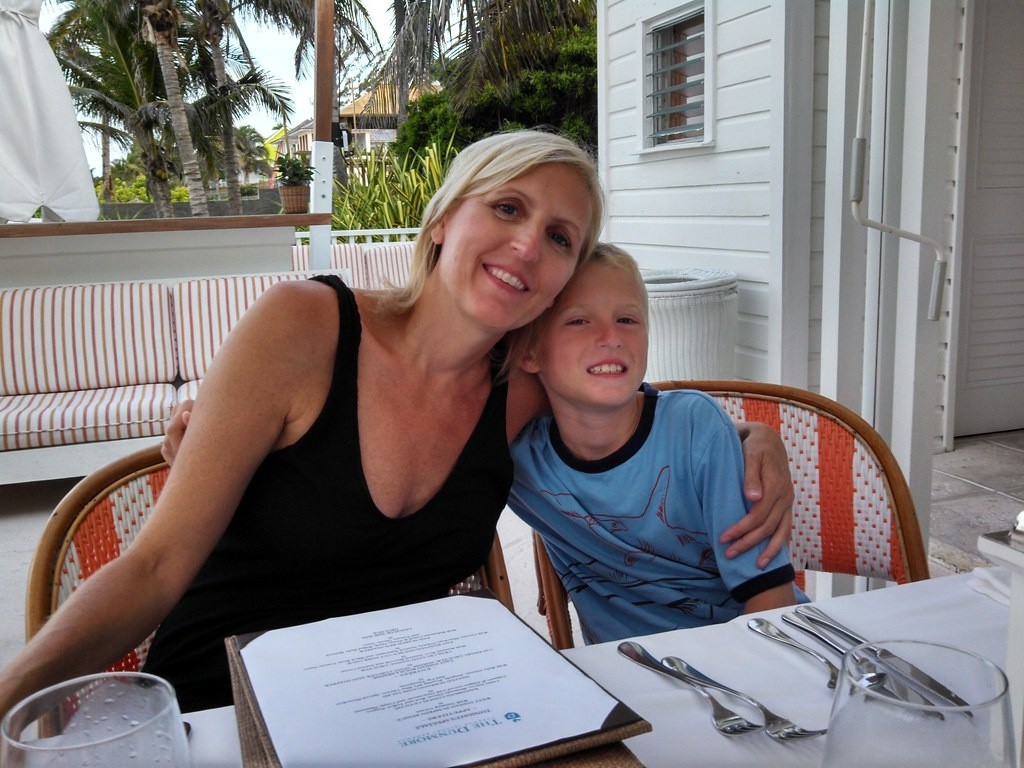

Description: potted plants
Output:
[271,149,319,215]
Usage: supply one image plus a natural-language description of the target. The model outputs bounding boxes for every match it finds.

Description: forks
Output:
[663,654,829,743]
[618,640,765,736]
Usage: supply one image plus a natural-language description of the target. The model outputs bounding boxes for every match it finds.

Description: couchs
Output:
[290,241,418,290]
[0,267,349,485]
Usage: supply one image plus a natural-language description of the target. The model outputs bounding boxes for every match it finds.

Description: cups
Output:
[825,640,1020,768]
[0,674,191,768]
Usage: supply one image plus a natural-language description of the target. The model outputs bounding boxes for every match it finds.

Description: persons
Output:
[0,130,810,721]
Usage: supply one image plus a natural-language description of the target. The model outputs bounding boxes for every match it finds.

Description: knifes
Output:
[796,604,974,719]
[782,612,945,721]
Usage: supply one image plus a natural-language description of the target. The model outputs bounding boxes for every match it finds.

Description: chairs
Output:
[531,380,931,649]
[26,445,514,741]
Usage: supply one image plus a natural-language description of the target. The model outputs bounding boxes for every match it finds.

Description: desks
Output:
[20,567,1024,767]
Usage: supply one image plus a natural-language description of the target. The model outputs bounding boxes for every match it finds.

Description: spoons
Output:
[748,616,889,691]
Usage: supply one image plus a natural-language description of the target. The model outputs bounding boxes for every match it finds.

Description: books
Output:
[225,590,654,767]
